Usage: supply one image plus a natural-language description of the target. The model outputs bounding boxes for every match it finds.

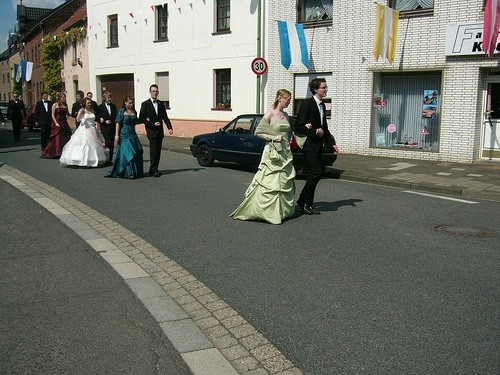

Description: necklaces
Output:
[274,109,285,119]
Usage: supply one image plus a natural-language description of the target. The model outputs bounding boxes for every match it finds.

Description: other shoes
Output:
[15,138,21,143]
[41,146,46,151]
[150,170,161,177]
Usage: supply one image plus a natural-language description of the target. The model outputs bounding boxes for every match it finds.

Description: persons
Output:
[87,93,100,123]
[35,92,72,160]
[105,95,150,179]
[7,93,27,142]
[58,96,110,168]
[95,91,119,166]
[0,108,6,127]
[229,88,309,226]
[292,78,339,216]
[72,90,85,129]
[139,84,174,177]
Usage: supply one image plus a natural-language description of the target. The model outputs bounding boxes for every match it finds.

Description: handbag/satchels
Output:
[269,137,280,161]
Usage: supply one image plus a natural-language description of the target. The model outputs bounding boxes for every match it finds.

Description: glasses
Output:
[150,91,159,92]
[14,96,18,97]
[319,85,328,89]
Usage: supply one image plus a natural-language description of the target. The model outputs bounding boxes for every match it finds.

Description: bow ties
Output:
[44,100,49,103]
[319,102,322,106]
[153,100,158,103]
[14,100,18,101]
[107,103,111,105]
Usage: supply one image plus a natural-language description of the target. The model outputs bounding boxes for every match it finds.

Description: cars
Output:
[190,114,338,179]
[21,103,40,131]
[0,100,9,116]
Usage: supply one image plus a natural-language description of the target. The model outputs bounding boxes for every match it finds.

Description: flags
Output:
[373,2,399,63]
[481,0,500,59]
[13,64,21,83]
[277,20,310,70]
[20,61,33,82]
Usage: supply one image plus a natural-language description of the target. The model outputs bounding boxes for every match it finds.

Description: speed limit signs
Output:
[251,57,268,75]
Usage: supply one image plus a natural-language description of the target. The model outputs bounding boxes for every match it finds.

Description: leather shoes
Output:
[308,205,321,215]
[296,200,313,215]
